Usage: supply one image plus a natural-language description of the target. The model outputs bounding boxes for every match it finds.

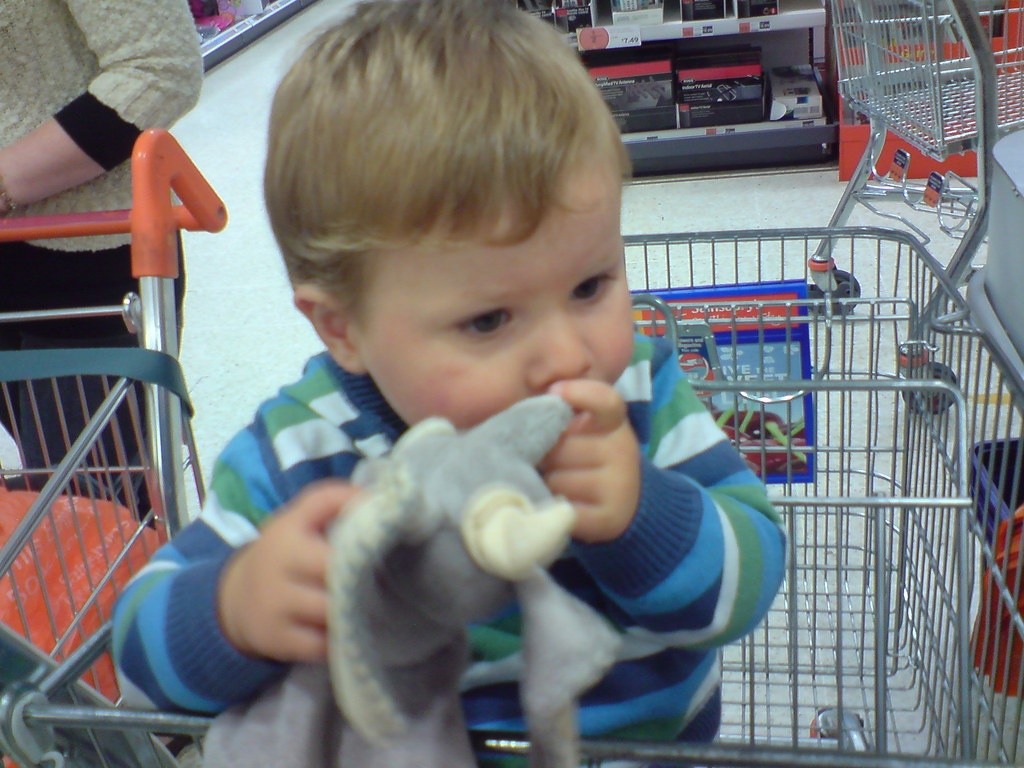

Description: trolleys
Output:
[0,128,1024,768]
[808,0,1024,413]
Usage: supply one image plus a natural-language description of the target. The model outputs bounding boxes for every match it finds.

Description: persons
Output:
[0,0,205,531]
[109,0,789,768]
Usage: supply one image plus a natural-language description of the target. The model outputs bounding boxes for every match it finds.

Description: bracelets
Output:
[0,177,16,211]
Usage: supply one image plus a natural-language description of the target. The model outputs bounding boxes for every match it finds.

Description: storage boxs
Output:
[674,48,766,128]
[577,51,677,132]
[770,63,823,120]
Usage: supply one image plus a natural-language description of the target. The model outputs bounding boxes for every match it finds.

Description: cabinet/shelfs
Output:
[505,0,841,180]
